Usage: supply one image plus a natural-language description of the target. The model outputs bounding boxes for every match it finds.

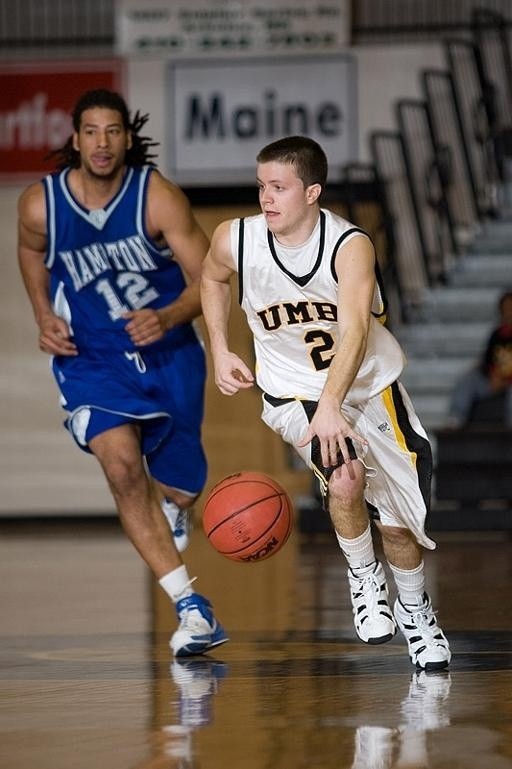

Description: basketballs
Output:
[202,471,293,563]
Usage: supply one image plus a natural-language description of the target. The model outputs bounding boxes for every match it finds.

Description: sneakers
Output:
[344,557,400,646]
[167,590,232,658]
[159,492,192,555]
[391,589,454,671]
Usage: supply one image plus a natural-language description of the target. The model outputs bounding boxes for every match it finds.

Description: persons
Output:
[18,87,230,660]
[200,135,454,671]
[450,287,512,426]
[353,664,451,767]
[160,655,227,733]
